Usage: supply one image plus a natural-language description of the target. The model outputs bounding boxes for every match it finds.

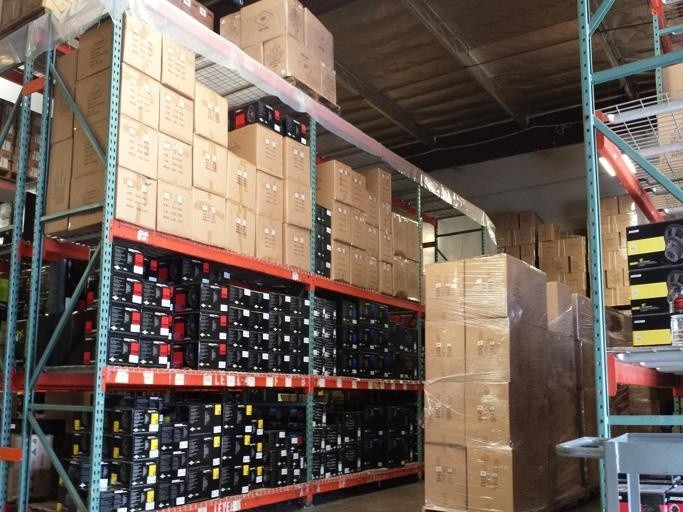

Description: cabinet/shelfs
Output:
[0,0,51,512]
[577,1,683,512]
[18,0,494,512]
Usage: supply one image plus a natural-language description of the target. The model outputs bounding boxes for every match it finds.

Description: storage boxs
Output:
[380,347,399,380]
[46,135,74,214]
[274,351,303,374]
[193,78,228,150]
[227,306,251,332]
[281,403,327,430]
[359,168,391,204]
[319,59,337,108]
[221,405,253,435]
[570,292,594,343]
[311,455,326,480]
[141,258,158,282]
[193,187,227,253]
[302,357,309,376]
[171,342,227,371]
[281,223,310,276]
[495,230,513,247]
[378,201,390,233]
[397,350,419,381]
[0,0,72,37]
[227,284,251,310]
[250,311,277,334]
[186,435,221,470]
[249,331,277,353]
[322,200,351,245]
[599,215,612,232]
[290,464,307,484]
[331,239,350,285]
[576,391,597,437]
[304,336,309,357]
[157,179,192,241]
[601,250,615,271]
[528,264,548,330]
[547,382,579,449]
[282,136,310,185]
[71,430,161,462]
[82,309,141,338]
[488,215,504,232]
[624,217,683,274]
[379,261,393,296]
[362,407,384,432]
[402,326,420,354]
[255,215,282,268]
[660,59,683,180]
[424,260,464,322]
[356,349,376,379]
[586,458,600,490]
[193,134,227,200]
[157,423,190,455]
[181,0,214,35]
[600,197,618,215]
[519,210,544,228]
[0,276,23,321]
[228,100,284,136]
[227,329,250,350]
[304,7,334,73]
[630,268,683,316]
[365,222,379,261]
[406,219,420,263]
[364,190,379,231]
[350,206,365,251]
[423,440,467,512]
[383,435,404,469]
[520,246,536,258]
[60,458,158,488]
[631,312,683,349]
[226,346,249,373]
[568,255,587,272]
[461,381,550,450]
[157,255,209,284]
[575,342,597,388]
[139,309,175,343]
[68,166,156,232]
[219,434,251,464]
[283,177,312,231]
[252,404,268,424]
[335,348,359,378]
[287,449,306,465]
[512,227,538,246]
[248,463,262,490]
[27,162,39,181]
[313,203,331,227]
[139,340,173,370]
[313,222,332,245]
[466,445,551,511]
[239,42,264,69]
[265,448,288,468]
[250,441,263,466]
[3,106,16,125]
[92,393,150,410]
[600,232,621,249]
[260,304,262,305]
[289,429,326,455]
[613,484,672,512]
[340,412,362,448]
[161,404,223,438]
[261,35,320,98]
[526,321,547,385]
[463,253,529,325]
[240,0,305,49]
[161,38,195,101]
[538,240,565,256]
[0,141,13,154]
[536,224,561,242]
[603,288,616,306]
[275,293,298,315]
[621,268,631,287]
[30,148,39,164]
[504,247,521,260]
[564,271,587,294]
[522,258,536,268]
[216,465,250,496]
[266,404,283,424]
[392,256,407,300]
[562,236,587,258]
[155,454,187,484]
[86,274,142,307]
[227,149,256,214]
[70,114,158,182]
[352,170,367,213]
[226,200,256,259]
[82,338,140,368]
[286,435,309,452]
[0,344,26,375]
[380,232,392,266]
[262,430,286,451]
[0,319,28,349]
[302,318,310,338]
[614,249,630,270]
[546,444,584,506]
[88,244,144,277]
[349,245,366,289]
[32,131,41,149]
[610,213,645,231]
[539,257,569,273]
[355,300,376,329]
[323,447,338,478]
[64,484,156,512]
[393,214,406,258]
[382,322,404,350]
[424,380,466,447]
[255,168,283,223]
[617,287,631,305]
[227,123,283,180]
[78,12,161,82]
[283,108,307,145]
[276,313,303,337]
[618,231,628,249]
[315,239,331,262]
[545,331,577,387]
[73,63,159,132]
[617,192,636,211]
[251,292,277,313]
[425,320,466,383]
[337,299,358,328]
[184,468,222,504]
[33,115,42,129]
[365,256,379,293]
[299,297,310,319]
[218,12,241,50]
[75,410,160,432]
[546,280,574,334]
[171,285,229,313]
[171,313,228,342]
[143,283,170,313]
[6,433,54,502]
[153,480,187,510]
[665,483,683,512]
[356,432,385,471]
[406,259,420,303]
[339,445,361,474]
[355,325,377,353]
[249,349,277,373]
[158,130,193,190]
[325,413,341,449]
[163,0,182,11]
[314,160,352,206]
[605,269,623,288]
[0,225,34,245]
[262,467,292,487]
[274,333,303,354]
[335,325,358,350]
[50,49,78,145]
[159,84,193,147]
[315,256,330,280]
[1,125,16,141]
[385,406,411,434]
[505,213,519,230]
[464,318,529,384]
[249,420,265,443]
[0,153,11,171]
[545,273,562,284]
